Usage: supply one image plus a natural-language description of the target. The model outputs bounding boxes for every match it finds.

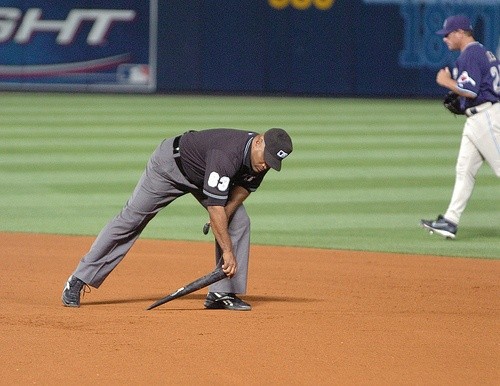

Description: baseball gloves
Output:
[442,90,464,114]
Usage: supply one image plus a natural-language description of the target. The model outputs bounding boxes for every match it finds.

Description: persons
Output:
[61,128,293,310]
[420,16,500,240]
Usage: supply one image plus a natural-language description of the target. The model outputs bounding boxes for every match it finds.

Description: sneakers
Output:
[420,215,456,239]
[204,292,251,311]
[61,275,91,307]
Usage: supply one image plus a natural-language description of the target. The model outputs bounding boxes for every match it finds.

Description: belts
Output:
[173,135,194,184]
[465,100,498,117]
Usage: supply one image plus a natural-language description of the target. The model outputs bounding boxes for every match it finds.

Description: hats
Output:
[264,128,292,172]
[435,15,472,34]
